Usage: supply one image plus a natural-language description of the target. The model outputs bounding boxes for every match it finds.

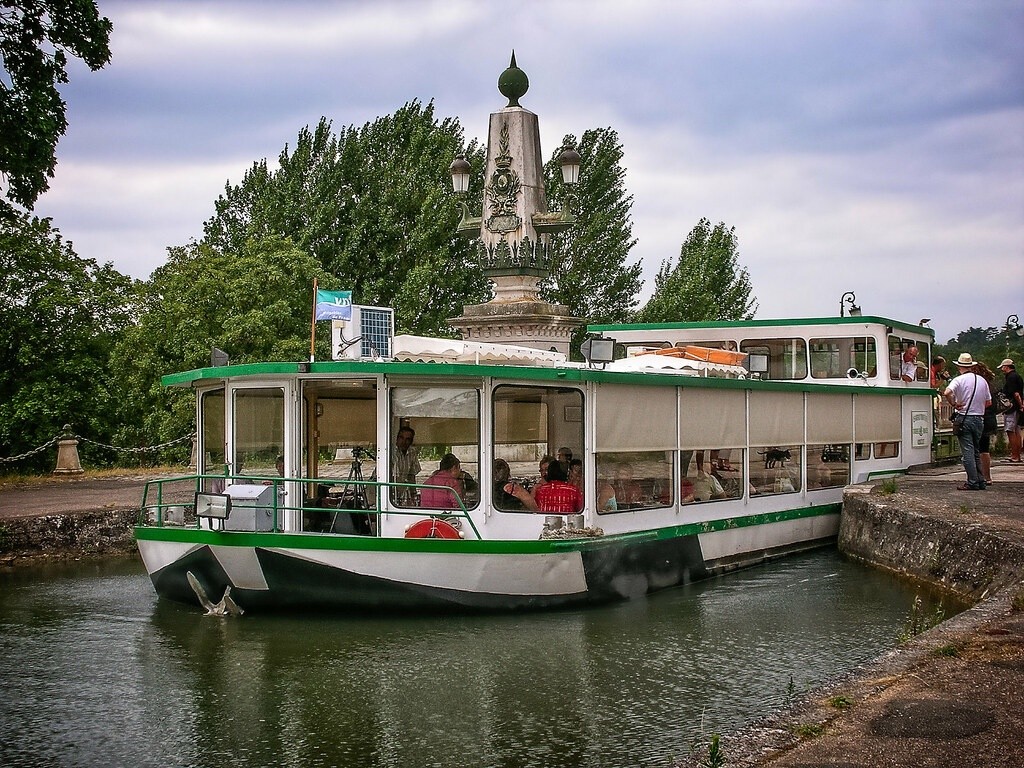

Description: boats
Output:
[133,314,936,613]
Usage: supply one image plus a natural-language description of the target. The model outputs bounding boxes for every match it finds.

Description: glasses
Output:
[539,468,549,472]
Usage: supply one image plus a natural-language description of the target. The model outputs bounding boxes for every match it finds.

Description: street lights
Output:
[1005,315,1024,359]
[840,292,862,317]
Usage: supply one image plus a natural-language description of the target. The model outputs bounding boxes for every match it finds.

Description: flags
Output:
[315,289,351,320]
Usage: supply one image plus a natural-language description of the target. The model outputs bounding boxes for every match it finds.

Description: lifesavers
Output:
[404,520,462,540]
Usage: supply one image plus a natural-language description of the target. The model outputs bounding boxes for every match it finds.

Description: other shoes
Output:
[957,482,987,491]
[986,479,993,485]
[710,471,721,478]
[1009,458,1023,463]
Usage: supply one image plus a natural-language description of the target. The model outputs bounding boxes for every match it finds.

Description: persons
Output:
[680,458,756,503]
[504,447,669,514]
[366,427,475,513]
[869,346,928,382]
[944,353,992,490]
[212,455,254,494]
[973,361,998,485]
[276,456,285,477]
[931,356,1024,463]
[807,449,832,490]
[681,449,736,478]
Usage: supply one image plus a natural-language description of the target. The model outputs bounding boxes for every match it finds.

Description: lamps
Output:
[742,354,770,380]
[211,347,228,367]
[193,491,232,530]
[580,338,616,369]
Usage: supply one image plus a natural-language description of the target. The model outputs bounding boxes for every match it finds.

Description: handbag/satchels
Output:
[953,421,964,435]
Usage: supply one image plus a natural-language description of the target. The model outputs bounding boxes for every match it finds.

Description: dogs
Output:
[757,449,792,469]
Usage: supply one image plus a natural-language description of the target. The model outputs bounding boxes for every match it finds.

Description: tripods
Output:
[330,445,376,537]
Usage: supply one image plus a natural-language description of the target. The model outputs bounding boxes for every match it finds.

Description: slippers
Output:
[717,465,735,471]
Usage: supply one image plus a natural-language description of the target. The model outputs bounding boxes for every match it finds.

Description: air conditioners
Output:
[332,305,394,361]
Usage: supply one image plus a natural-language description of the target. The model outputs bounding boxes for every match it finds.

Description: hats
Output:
[917,361,929,369]
[996,359,1014,368]
[952,352,978,367]
